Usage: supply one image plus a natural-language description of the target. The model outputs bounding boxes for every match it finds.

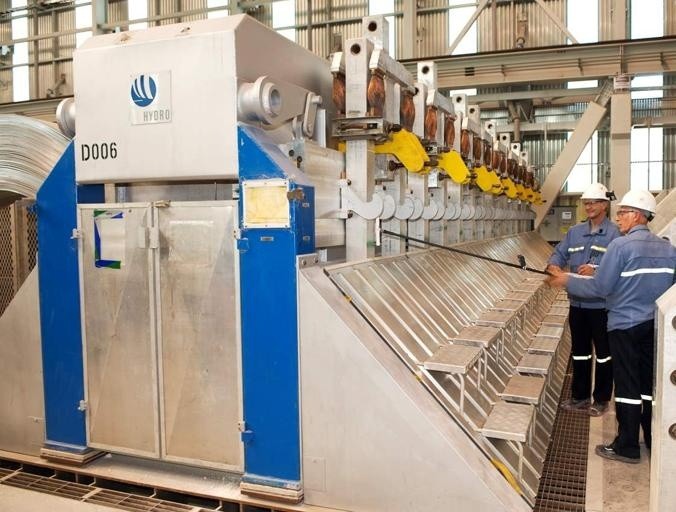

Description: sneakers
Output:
[595,445,640,464]
[560,399,609,416]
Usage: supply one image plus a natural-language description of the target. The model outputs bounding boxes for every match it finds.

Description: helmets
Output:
[617,188,657,216]
[580,183,611,203]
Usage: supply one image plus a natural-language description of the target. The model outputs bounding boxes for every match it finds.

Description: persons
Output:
[545,183,676,464]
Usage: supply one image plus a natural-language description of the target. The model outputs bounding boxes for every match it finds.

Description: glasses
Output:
[584,201,604,205]
[617,211,635,215]
